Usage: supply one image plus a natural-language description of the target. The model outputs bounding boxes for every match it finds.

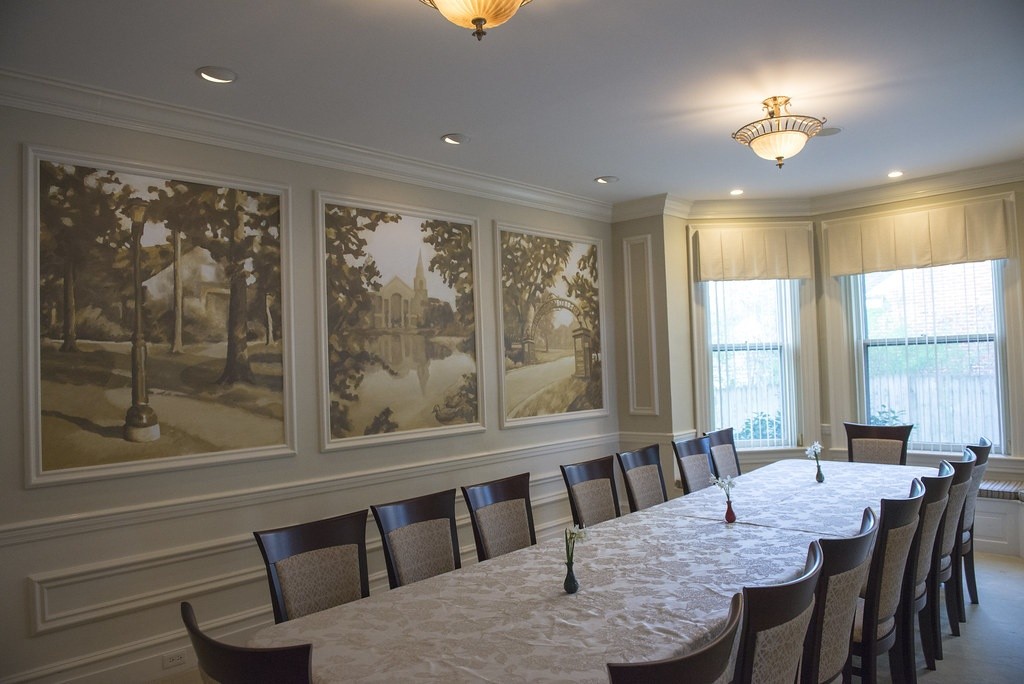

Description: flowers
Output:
[709,473,736,501]
[805,441,824,465]
[565,522,586,562]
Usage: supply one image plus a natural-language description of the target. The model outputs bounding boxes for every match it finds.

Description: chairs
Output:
[559,422,992,683]
[252,509,370,625]
[371,488,461,590]
[460,472,536,563]
[181,602,313,684]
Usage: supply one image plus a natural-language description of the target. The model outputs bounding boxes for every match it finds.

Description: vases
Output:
[725,501,736,523]
[564,562,578,593]
[816,465,824,482]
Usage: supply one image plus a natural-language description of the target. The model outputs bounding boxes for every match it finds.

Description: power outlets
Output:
[162,649,186,669]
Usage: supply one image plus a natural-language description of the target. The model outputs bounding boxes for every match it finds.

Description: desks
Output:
[248,459,940,683]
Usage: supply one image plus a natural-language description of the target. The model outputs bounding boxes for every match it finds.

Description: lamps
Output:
[420,0,533,40]
[732,96,827,170]
